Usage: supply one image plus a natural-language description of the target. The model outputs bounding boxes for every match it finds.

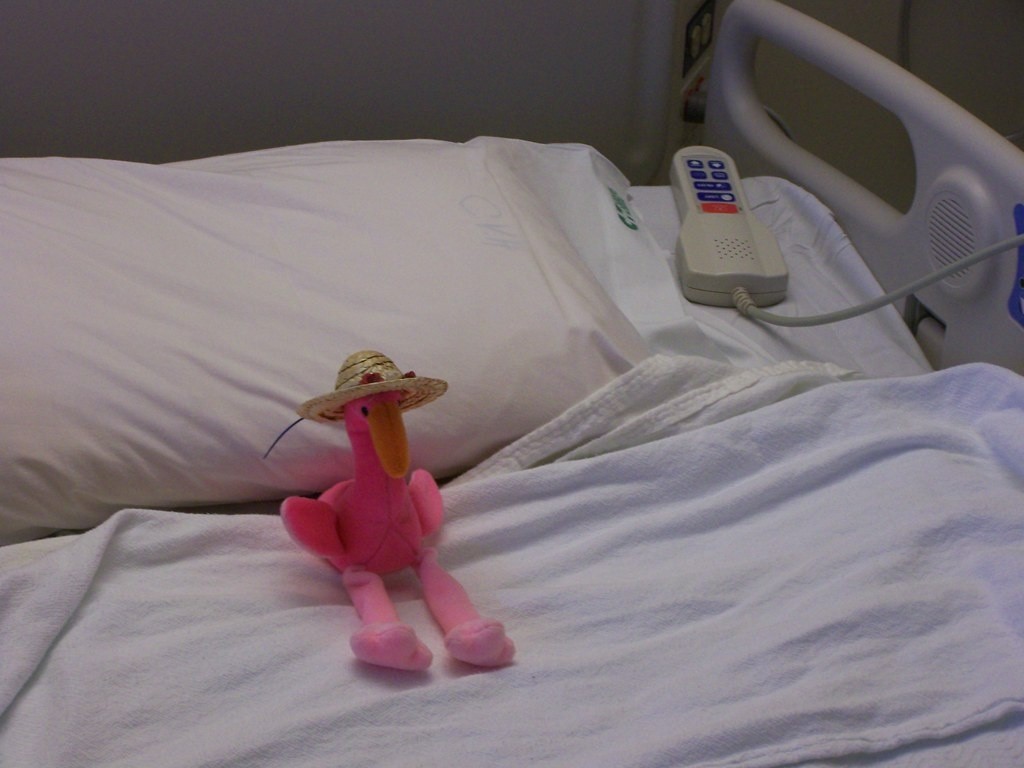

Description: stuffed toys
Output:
[280,350,515,671]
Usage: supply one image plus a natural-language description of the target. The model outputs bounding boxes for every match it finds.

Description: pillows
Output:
[0,134,629,545]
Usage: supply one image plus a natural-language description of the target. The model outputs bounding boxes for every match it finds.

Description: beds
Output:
[0,1,1024,767]
